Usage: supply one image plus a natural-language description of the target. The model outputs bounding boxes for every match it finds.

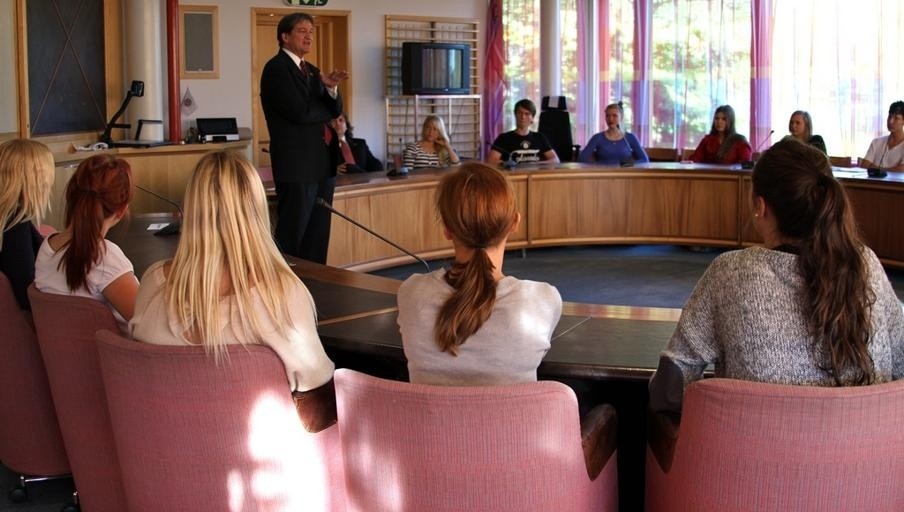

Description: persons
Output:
[860,101,902,172]
[403,113,459,173]
[788,111,827,156]
[645,135,903,418]
[124,150,334,394]
[0,138,57,314]
[394,161,564,389]
[579,104,649,164]
[258,12,349,265]
[680,105,755,166]
[483,99,561,167]
[329,107,387,177]
[33,154,142,337]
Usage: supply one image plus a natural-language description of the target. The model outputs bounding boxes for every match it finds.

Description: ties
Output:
[300,61,332,146]
[340,139,355,165]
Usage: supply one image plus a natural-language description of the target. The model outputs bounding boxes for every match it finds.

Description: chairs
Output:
[24,280,128,512]
[94,329,339,512]
[828,156,852,168]
[0,274,80,512]
[681,148,695,161]
[330,368,621,512]
[639,378,904,511]
[393,154,402,170]
[537,94,582,164]
[639,148,678,161]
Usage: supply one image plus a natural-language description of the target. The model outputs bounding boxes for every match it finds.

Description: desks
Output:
[105,165,903,390]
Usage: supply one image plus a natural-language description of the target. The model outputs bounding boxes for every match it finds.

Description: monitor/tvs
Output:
[402,43,470,95]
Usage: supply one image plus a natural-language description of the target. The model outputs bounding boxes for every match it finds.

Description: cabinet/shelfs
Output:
[381,92,485,170]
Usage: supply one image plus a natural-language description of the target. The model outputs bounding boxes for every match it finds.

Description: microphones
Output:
[314,194,431,275]
[132,182,183,237]
[261,147,270,154]
[742,130,774,169]
[616,123,634,167]
[868,131,895,178]
[485,141,516,169]
[387,138,408,176]
